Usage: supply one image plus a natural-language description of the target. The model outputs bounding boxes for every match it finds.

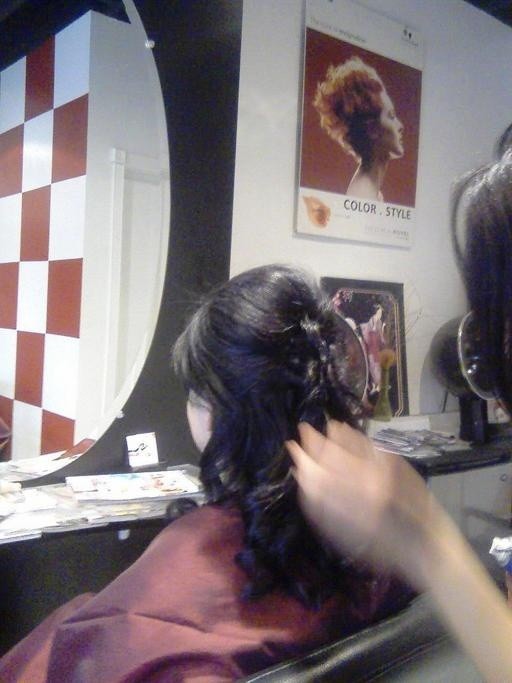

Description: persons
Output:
[310,51,406,203]
[288,124,512,683]
[1,266,416,683]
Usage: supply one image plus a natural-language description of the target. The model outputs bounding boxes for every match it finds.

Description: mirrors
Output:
[0,0,172,483]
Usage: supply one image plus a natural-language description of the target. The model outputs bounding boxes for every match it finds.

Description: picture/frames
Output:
[318,275,410,417]
